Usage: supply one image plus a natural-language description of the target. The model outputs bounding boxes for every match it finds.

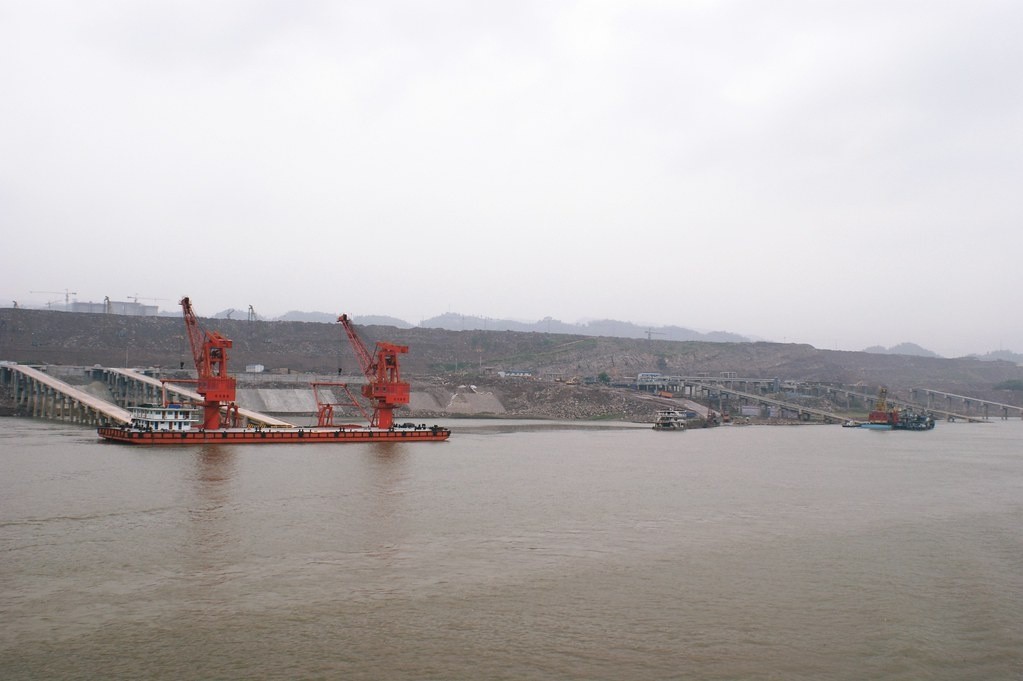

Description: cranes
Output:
[29,287,78,303]
[644,328,667,339]
[126,293,168,306]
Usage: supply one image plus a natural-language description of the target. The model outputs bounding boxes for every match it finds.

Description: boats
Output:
[652,408,688,431]
[840,387,935,431]
[97,296,451,444]
